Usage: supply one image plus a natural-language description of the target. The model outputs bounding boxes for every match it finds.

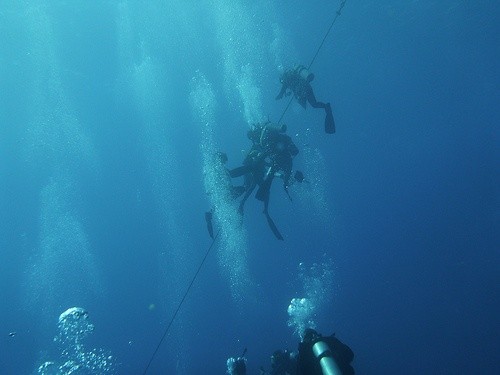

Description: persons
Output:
[275,70,337,135]
[227,328,354,375]
[205,121,299,241]
